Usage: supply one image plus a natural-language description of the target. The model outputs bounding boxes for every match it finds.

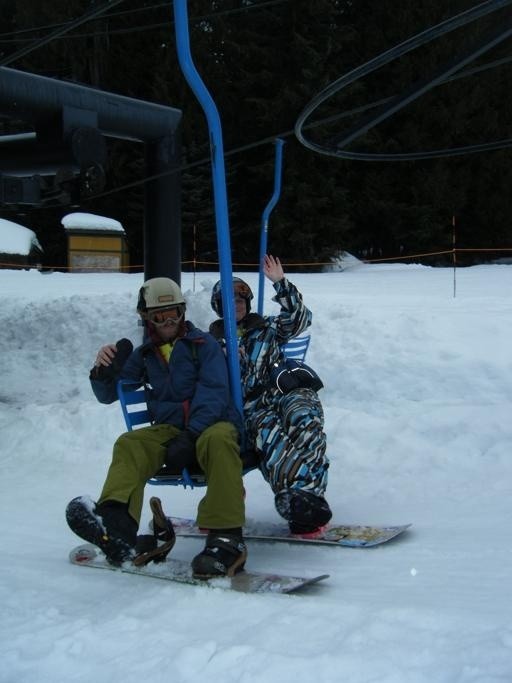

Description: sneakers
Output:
[274,487,332,534]
[191,531,248,578]
[65,494,139,568]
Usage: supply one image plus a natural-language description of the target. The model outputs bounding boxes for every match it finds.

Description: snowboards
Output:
[69,542,330,595]
[148,513,411,550]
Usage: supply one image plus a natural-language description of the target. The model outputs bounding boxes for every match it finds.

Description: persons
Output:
[64,273,248,581]
[203,254,334,535]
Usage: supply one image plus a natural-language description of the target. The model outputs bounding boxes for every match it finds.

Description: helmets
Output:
[211,277,253,316]
[136,277,186,312]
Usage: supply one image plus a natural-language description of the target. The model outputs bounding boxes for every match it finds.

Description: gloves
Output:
[160,429,195,471]
[89,338,133,386]
[266,351,324,394]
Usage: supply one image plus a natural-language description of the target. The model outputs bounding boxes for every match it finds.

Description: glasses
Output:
[139,302,186,327]
